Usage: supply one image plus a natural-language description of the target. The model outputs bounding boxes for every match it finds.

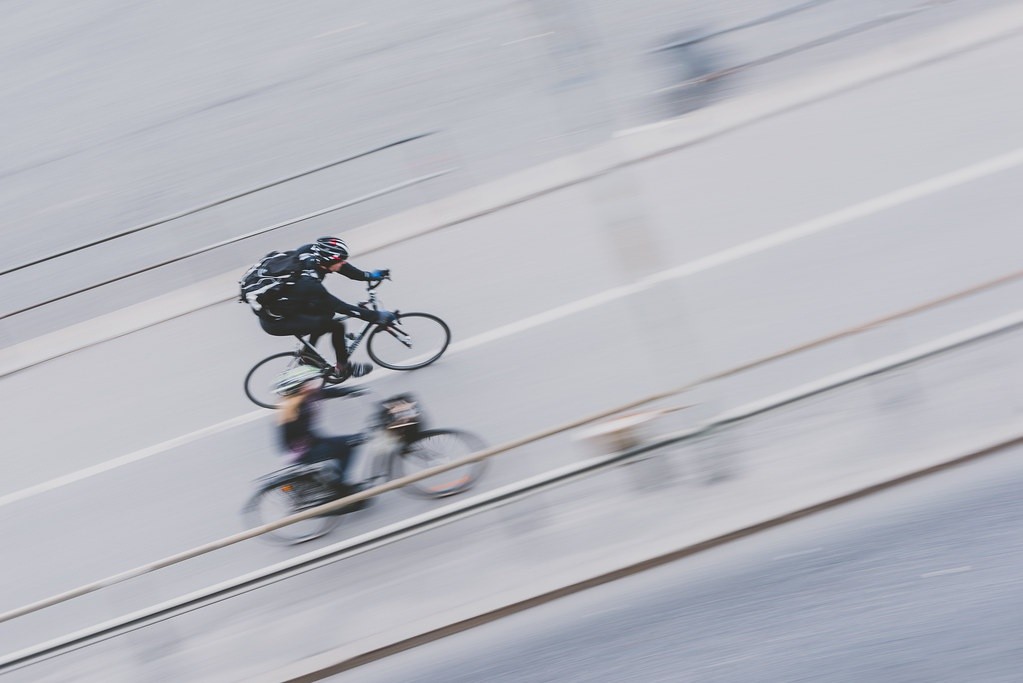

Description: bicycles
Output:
[243,268,452,413]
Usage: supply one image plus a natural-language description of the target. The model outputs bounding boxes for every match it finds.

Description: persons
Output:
[272,366,399,518]
[238,235,396,378]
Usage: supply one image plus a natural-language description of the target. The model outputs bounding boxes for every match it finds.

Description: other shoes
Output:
[300,353,331,368]
[337,363,373,377]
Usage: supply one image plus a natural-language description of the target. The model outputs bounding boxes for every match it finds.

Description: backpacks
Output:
[240,249,321,321]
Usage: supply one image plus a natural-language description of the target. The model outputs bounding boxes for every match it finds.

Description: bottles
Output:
[345,332,356,349]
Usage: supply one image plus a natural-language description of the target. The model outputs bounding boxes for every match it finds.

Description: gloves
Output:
[370,269,389,279]
[374,311,396,325]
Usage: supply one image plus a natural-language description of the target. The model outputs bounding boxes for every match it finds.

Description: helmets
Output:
[317,237,349,261]
